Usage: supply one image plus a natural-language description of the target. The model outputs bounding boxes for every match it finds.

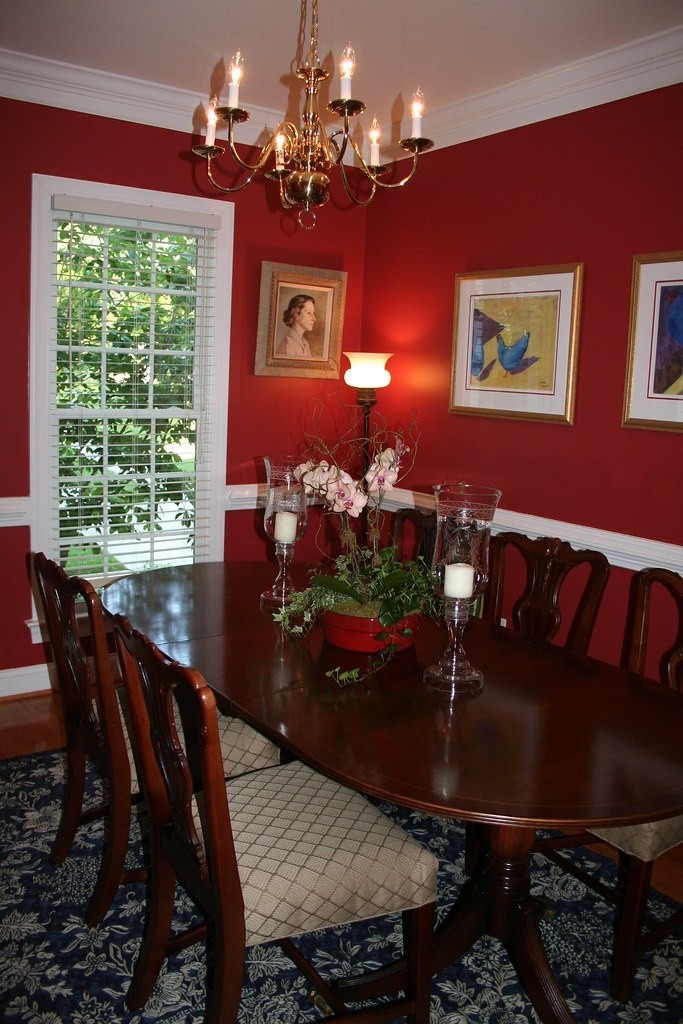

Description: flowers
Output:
[268,412,485,691]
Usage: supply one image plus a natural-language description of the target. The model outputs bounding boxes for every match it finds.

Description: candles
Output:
[272,509,298,544]
[445,563,475,599]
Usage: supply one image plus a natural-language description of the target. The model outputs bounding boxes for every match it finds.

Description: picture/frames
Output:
[254,261,348,377]
[619,250,683,433]
[448,262,586,425]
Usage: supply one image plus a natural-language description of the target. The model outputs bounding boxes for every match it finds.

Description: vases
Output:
[322,606,423,651]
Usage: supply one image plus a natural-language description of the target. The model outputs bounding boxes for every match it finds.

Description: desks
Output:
[101,559,683,1024]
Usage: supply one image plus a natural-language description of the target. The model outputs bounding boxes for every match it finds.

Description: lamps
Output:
[190,0,435,231]
[343,351,394,572]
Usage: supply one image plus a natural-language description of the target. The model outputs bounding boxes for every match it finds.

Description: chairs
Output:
[391,508,492,616]
[489,531,612,656]
[22,549,441,1024]
[624,567,683,690]
